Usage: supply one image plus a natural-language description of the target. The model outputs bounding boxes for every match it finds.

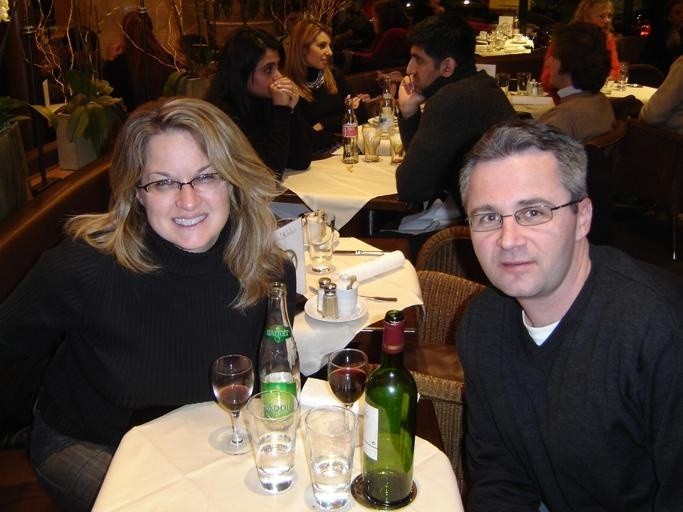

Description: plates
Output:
[304,295,370,323]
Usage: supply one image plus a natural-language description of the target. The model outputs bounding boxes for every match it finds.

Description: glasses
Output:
[465,198,581,233]
[136,172,222,195]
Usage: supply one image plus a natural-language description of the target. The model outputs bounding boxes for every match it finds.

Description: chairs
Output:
[344,63,407,95]
[0,156,114,307]
[616,35,644,62]
[625,118,682,262]
[417,226,486,283]
[627,63,664,87]
[417,270,487,344]
[371,363,467,499]
[584,120,626,200]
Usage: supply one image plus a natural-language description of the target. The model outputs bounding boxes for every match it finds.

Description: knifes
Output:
[359,295,398,302]
[334,249,384,256]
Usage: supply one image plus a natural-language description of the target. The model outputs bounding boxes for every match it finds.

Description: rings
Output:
[292,86,298,93]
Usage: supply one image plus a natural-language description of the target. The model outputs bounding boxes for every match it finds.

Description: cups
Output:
[246,390,300,492]
[616,60,628,92]
[306,214,338,274]
[516,72,531,96]
[335,280,359,317]
[490,72,511,97]
[362,122,383,163]
[387,125,408,165]
[495,33,505,49]
[487,36,492,51]
[305,405,356,510]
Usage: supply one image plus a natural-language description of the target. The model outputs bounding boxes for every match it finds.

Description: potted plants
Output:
[53,71,128,174]
[162,63,215,99]
[0,93,58,219]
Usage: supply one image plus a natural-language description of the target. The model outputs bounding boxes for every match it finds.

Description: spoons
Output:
[346,274,357,290]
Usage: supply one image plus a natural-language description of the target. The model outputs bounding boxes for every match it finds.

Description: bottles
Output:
[513,13,521,37]
[342,99,359,164]
[538,82,544,96]
[379,74,395,122]
[259,282,302,429]
[530,81,539,95]
[360,311,419,505]
[323,283,338,318]
[317,278,331,313]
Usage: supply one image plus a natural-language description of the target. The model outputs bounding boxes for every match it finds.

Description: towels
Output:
[299,376,421,416]
[338,247,405,285]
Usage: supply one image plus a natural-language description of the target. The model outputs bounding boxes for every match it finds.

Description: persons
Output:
[394,13,521,217]
[285,18,368,145]
[531,22,617,174]
[203,27,316,193]
[574,0,620,83]
[103,10,174,110]
[0,94,297,511]
[639,55,683,136]
[164,32,212,96]
[453,119,683,512]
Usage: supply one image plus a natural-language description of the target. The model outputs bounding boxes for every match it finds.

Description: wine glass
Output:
[211,355,255,456]
[329,348,371,447]
